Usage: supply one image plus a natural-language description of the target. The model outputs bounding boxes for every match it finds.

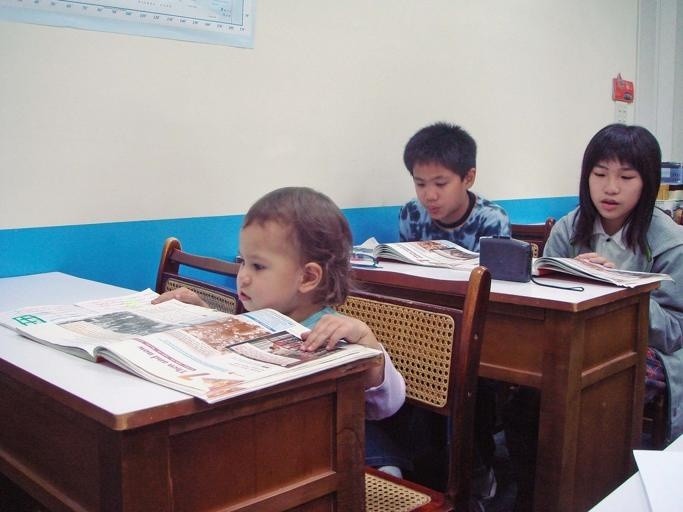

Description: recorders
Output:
[479,234,532,283]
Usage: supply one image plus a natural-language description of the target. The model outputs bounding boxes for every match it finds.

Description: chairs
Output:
[326,262,491,511]
[153,235,250,317]
[640,208,682,447]
[499,217,555,258]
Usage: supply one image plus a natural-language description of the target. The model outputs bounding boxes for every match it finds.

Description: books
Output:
[655,180,682,200]
[529,253,674,290]
[351,236,479,271]
[1,287,379,405]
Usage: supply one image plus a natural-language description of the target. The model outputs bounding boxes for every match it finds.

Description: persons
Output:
[542,126,682,442]
[398,123,512,252]
[151,187,406,420]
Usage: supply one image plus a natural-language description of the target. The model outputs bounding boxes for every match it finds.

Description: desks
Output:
[347,240,661,512]
[0,269,386,512]
[586,432,683,512]
